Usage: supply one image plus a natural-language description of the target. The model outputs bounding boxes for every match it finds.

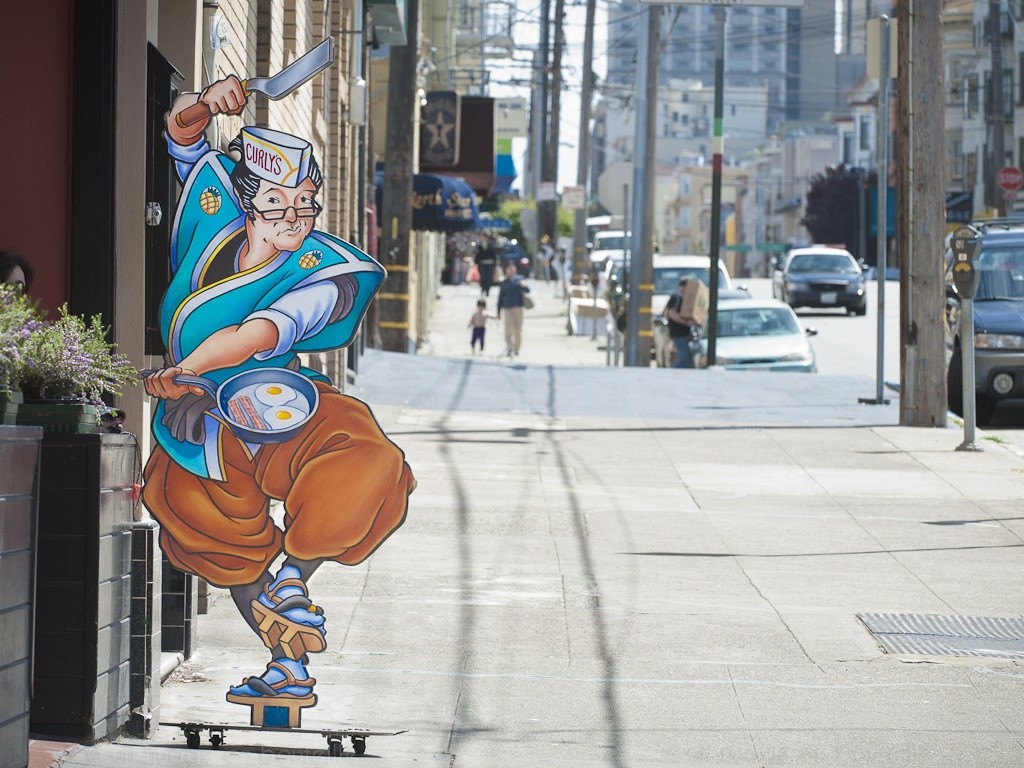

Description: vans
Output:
[649,255,732,350]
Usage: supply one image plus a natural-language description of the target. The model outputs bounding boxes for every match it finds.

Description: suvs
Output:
[945,219,1023,417]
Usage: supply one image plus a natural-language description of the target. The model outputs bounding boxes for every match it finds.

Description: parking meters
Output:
[590,264,601,342]
[606,277,624,367]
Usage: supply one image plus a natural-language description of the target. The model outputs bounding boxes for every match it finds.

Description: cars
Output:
[770,243,869,316]
[706,285,754,302]
[586,229,631,331]
[690,298,820,375]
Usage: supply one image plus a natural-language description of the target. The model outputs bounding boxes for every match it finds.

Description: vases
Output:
[18,401,100,437]
[0,384,25,427]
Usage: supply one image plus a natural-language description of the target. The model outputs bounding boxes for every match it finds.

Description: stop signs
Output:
[999,167,1022,189]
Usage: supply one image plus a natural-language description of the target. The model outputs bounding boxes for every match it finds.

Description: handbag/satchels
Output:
[522,294,535,308]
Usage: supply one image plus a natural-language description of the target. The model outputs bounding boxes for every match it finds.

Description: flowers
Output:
[0,284,64,405]
[19,300,139,426]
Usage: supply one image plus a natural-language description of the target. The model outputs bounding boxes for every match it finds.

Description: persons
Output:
[665,274,698,368]
[457,231,559,356]
[0,249,37,302]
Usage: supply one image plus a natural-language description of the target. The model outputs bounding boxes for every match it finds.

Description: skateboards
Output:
[159,720,409,757]
